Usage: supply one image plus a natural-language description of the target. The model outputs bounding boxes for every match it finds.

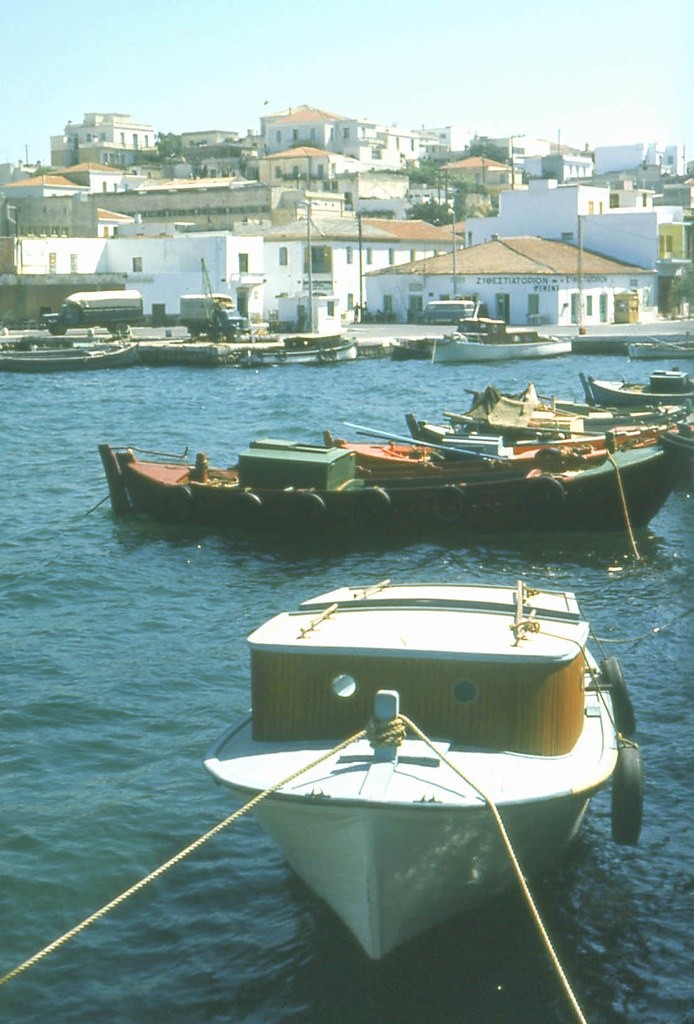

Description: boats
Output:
[626,331,693,362]
[0,315,574,373]
[201,578,646,963]
[83,366,694,533]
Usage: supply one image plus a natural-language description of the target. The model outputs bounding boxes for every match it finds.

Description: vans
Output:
[418,300,476,324]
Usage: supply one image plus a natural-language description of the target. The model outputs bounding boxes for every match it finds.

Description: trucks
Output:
[42,289,147,338]
[180,294,252,337]
